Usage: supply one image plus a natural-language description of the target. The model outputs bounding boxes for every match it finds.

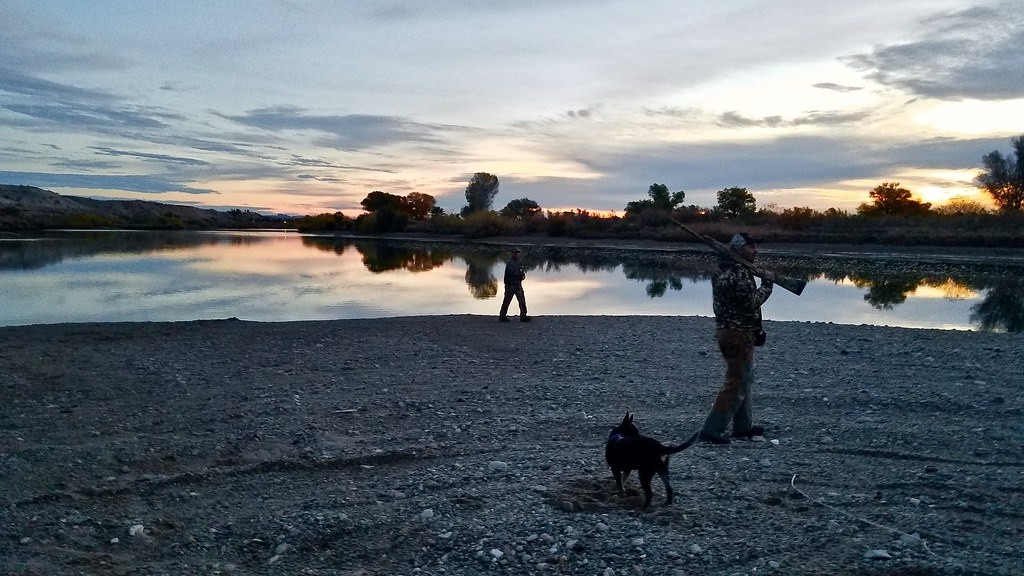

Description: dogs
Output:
[605,410,702,511]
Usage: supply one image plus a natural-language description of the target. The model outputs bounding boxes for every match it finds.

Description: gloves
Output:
[761,270,776,288]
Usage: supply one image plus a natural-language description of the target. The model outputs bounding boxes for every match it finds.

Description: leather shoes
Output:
[700,432,731,444]
[731,426,764,437]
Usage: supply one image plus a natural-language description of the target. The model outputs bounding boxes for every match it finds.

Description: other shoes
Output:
[500,318,510,322]
[521,317,532,322]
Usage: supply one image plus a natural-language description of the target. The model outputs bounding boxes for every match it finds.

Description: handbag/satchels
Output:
[753,329,766,347]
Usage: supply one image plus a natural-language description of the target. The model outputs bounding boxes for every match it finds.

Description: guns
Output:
[656,207,807,297]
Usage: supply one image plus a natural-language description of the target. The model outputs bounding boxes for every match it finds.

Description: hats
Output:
[511,247,521,252]
[731,232,763,252]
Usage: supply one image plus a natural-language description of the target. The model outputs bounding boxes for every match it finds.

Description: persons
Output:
[698,232,774,444]
[499,247,533,323]
